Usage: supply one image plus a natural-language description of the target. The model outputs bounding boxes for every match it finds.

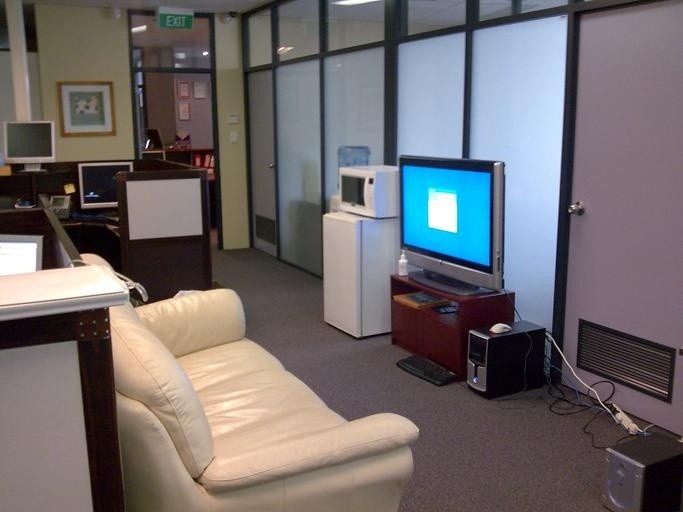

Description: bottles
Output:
[397,248,408,275]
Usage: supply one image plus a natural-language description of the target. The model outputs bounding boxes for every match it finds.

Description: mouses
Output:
[489,323,512,334]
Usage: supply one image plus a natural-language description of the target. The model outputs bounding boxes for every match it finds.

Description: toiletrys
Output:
[396,248,408,278]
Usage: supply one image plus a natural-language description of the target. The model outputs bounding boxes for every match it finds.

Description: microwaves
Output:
[338,165,400,219]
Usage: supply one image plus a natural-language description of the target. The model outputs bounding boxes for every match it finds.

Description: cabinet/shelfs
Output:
[318,208,402,339]
[383,270,516,377]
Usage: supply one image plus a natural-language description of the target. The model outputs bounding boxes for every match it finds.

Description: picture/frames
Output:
[54,78,115,139]
[176,80,191,100]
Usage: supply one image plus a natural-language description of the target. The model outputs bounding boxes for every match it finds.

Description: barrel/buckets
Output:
[336,144,371,196]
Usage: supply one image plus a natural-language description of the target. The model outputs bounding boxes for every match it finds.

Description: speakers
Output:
[600,432,683,512]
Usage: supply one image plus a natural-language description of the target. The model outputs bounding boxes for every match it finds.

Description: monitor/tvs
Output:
[400,153,505,296]
[78,162,134,217]
[0,233,46,275]
[3,121,56,172]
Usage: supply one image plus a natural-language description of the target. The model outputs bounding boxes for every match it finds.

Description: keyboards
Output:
[396,354,458,387]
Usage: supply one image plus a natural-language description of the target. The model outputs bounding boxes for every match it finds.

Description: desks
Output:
[141,144,218,231]
[0,157,214,306]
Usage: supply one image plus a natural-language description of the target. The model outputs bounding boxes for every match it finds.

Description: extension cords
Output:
[611,404,638,435]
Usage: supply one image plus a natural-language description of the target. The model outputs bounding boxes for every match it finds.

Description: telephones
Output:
[48,195,71,219]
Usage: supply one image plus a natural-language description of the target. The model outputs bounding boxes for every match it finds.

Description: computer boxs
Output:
[468,320,546,400]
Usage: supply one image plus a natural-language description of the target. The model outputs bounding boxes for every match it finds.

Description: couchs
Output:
[78,245,421,512]
